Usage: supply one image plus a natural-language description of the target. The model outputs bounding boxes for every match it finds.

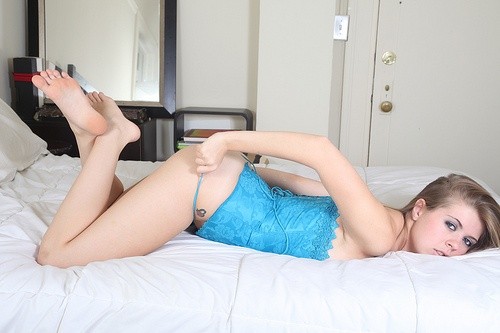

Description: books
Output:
[177,129,240,146]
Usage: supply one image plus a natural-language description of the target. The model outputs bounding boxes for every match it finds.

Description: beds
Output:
[0,97,500,333]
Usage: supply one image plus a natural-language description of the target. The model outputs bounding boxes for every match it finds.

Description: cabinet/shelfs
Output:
[171,107,253,159]
[46,100,172,162]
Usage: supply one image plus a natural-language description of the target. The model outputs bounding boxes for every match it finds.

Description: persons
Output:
[32,68,500,269]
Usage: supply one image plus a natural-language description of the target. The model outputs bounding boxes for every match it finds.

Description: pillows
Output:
[0,98,47,187]
